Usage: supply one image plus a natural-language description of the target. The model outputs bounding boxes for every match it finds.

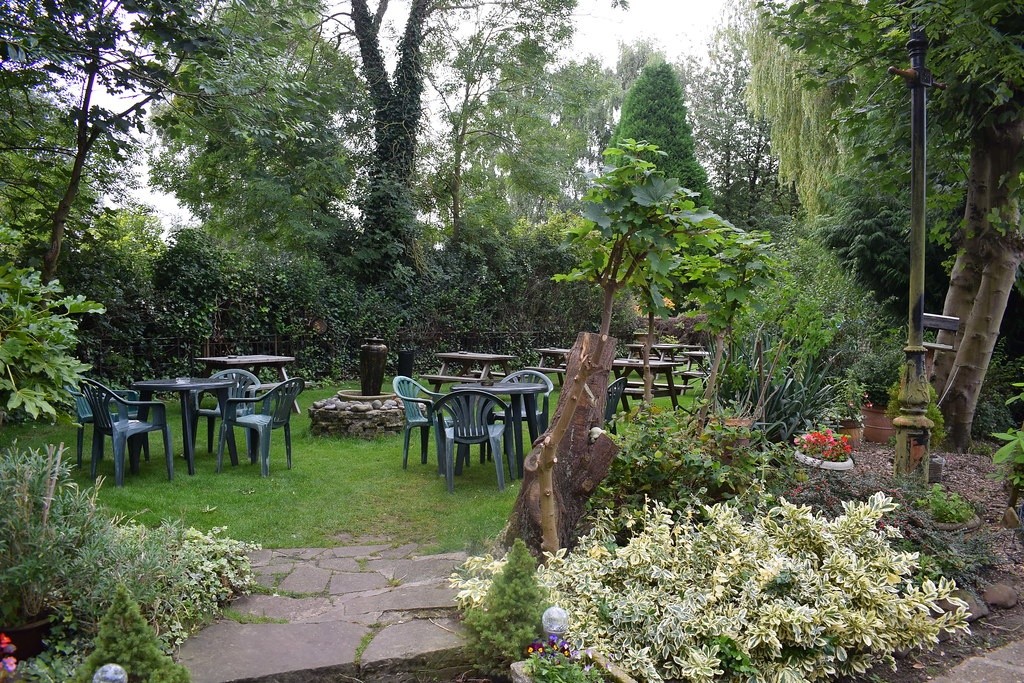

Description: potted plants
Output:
[909,482,980,544]
[0,439,127,663]
[825,347,946,482]
[708,357,754,455]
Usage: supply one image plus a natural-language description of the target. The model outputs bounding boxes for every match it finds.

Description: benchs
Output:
[607,355,712,400]
[238,381,313,393]
[525,364,568,374]
[419,371,529,384]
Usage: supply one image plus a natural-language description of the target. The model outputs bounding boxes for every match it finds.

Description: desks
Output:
[534,348,571,386]
[679,351,712,396]
[450,383,548,479]
[129,378,239,474]
[194,354,301,415]
[613,360,684,413]
[429,350,518,400]
[652,344,705,360]
[624,344,680,380]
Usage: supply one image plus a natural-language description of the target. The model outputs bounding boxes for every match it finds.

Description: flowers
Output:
[524,634,614,683]
[792,423,852,462]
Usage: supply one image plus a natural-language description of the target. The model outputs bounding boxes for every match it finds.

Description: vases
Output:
[794,450,853,477]
[510,648,639,683]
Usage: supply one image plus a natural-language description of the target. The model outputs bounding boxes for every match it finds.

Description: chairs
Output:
[605,377,628,435]
[392,375,471,473]
[186,369,261,458]
[214,376,305,478]
[431,390,514,492]
[485,369,554,462]
[78,378,175,487]
[64,374,150,471]
